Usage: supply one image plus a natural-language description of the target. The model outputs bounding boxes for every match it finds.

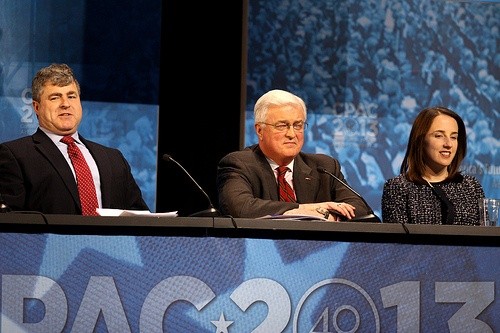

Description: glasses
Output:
[258,122,308,131]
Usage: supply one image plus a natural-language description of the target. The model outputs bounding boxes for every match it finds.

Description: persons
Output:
[246,0,500,209]
[382,107,491,225]
[0,65,151,216]
[219,90,377,222]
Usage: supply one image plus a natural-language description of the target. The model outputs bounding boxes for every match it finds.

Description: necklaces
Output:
[423,175,436,187]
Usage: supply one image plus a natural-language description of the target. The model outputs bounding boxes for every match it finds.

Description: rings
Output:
[337,204,342,206]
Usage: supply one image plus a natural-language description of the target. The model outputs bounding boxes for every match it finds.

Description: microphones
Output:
[163,154,222,217]
[317,166,381,221]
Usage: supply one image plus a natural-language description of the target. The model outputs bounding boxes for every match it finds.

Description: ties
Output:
[276,166,295,203]
[60,135,99,216]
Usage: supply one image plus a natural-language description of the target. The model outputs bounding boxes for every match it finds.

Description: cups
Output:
[477,198,500,227]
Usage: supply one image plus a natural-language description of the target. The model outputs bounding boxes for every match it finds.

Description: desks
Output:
[0,212,500,333]
